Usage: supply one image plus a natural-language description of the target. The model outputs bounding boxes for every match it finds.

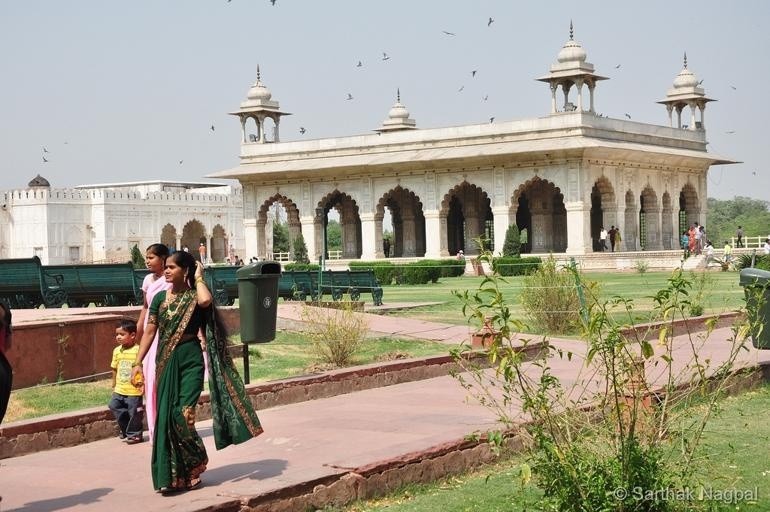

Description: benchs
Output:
[0,251,386,311]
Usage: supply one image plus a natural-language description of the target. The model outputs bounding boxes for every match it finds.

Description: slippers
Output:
[156,487,184,493]
[186,477,202,491]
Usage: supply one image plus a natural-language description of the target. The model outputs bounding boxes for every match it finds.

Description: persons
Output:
[764,239,770,254]
[682,222,744,267]
[383,238,390,258]
[0,297,12,500]
[107,243,230,492]
[226,256,260,265]
[457,250,465,260]
[598,224,622,252]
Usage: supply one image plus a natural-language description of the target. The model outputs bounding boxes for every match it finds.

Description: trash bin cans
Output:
[235,261,283,344]
[739,267,770,349]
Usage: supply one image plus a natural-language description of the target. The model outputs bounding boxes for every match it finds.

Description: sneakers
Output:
[126,435,144,444]
